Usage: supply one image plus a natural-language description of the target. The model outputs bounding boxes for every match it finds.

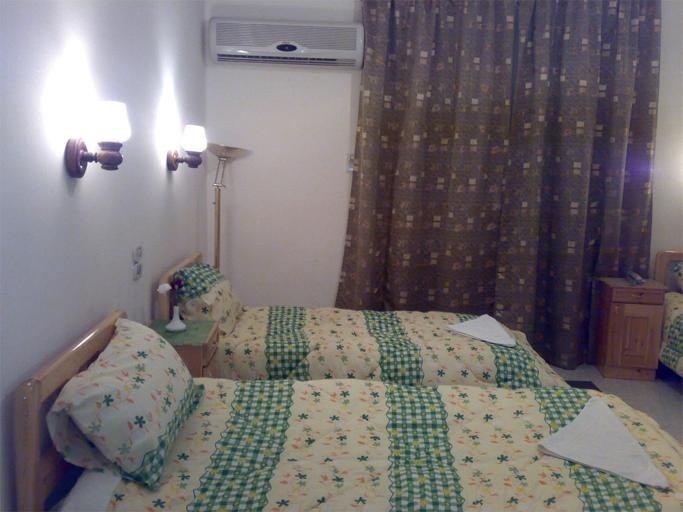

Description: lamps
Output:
[58,93,132,180]
[206,141,249,270]
[162,122,210,175]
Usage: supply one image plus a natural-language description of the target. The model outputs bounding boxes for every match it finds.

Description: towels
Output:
[532,394,671,493]
[443,311,517,351]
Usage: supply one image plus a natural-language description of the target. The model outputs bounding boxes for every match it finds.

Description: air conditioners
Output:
[205,14,368,71]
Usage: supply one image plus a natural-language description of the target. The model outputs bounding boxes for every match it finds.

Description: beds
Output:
[10,305,681,511]
[144,251,571,390]
[650,248,681,380]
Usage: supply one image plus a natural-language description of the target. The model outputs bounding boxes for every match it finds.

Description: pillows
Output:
[173,261,246,336]
[45,314,204,491]
[668,258,682,296]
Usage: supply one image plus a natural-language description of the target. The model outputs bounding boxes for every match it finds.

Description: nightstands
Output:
[145,316,222,378]
[590,270,668,383]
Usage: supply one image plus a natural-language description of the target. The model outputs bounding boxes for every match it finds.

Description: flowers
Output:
[156,278,186,307]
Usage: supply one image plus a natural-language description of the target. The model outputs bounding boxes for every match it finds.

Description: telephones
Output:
[626,271,645,284]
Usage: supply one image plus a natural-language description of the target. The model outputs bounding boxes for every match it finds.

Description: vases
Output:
[164,302,186,334]
